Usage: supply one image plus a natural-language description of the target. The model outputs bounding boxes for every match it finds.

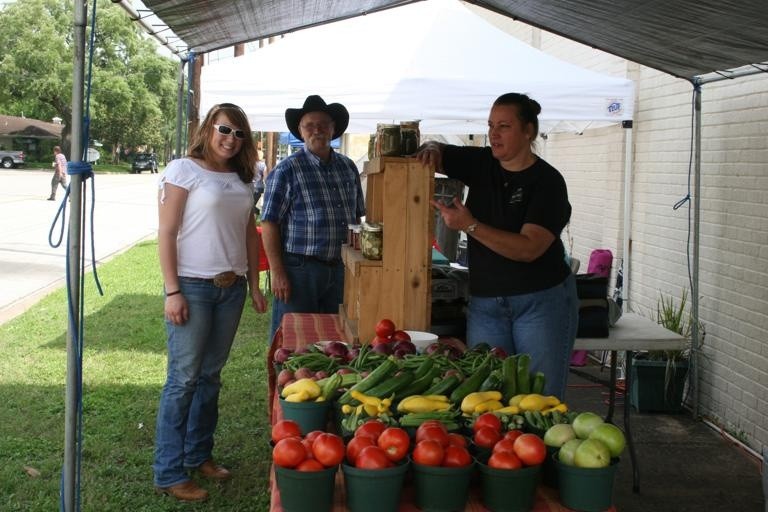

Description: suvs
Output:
[132,154,158,174]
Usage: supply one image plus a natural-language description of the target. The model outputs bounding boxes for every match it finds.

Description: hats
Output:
[285,96,349,141]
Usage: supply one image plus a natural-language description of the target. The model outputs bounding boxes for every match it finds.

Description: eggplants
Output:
[282,378,568,417]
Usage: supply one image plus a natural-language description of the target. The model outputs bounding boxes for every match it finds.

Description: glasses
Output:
[213,124,246,140]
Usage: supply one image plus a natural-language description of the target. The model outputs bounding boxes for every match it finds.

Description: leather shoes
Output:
[200,460,231,479]
[156,481,208,502]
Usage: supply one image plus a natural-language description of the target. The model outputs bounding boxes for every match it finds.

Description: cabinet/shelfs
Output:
[340,157,434,348]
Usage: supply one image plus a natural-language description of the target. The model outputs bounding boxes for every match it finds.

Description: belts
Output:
[214,271,237,288]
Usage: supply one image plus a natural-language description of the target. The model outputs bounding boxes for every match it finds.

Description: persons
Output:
[153,103,269,501]
[415,93,580,402]
[48,145,71,201]
[259,94,366,346]
[253,150,268,220]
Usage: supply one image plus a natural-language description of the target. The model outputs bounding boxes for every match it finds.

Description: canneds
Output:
[360,223,382,260]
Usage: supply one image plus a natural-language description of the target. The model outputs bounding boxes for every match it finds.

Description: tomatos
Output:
[371,319,412,347]
[271,412,545,470]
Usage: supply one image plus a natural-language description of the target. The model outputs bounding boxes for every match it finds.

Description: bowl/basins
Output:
[403,330,439,354]
[314,341,348,354]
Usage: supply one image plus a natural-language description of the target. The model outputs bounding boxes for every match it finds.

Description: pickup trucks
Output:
[0,150,25,168]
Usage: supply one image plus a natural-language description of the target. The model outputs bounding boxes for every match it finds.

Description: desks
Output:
[573,308,686,495]
[268,313,619,512]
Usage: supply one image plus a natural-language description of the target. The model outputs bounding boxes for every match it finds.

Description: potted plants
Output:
[625,286,711,413]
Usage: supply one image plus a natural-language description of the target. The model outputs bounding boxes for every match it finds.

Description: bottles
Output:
[367,120,420,161]
[348,222,383,261]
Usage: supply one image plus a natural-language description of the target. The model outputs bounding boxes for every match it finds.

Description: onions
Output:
[543,412,626,468]
[273,341,505,387]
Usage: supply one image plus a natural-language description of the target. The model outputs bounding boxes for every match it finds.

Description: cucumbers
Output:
[322,353,546,406]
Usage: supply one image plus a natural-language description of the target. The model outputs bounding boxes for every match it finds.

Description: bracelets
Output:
[167,290,181,296]
[466,222,479,234]
